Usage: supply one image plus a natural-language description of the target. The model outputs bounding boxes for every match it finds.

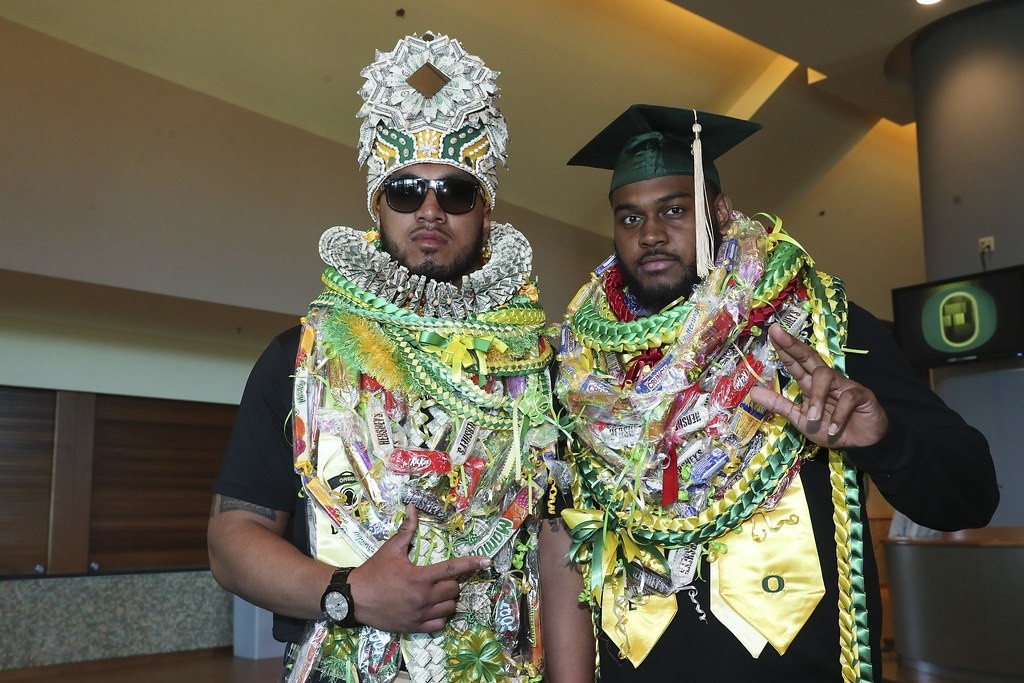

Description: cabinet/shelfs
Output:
[0,385,239,580]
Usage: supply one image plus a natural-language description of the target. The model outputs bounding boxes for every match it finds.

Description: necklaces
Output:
[554,210,874,683]
[304,215,554,434]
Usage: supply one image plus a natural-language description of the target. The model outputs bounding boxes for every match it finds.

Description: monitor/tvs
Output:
[892,263,1024,368]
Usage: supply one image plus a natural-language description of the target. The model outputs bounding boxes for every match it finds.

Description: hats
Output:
[567,104,763,281]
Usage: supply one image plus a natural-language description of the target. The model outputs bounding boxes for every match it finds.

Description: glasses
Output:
[376,173,487,214]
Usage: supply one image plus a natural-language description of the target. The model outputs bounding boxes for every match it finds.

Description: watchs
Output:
[320,567,364,627]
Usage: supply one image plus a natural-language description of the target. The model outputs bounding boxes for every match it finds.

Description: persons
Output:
[556,102,1003,683]
[207,28,598,683]
